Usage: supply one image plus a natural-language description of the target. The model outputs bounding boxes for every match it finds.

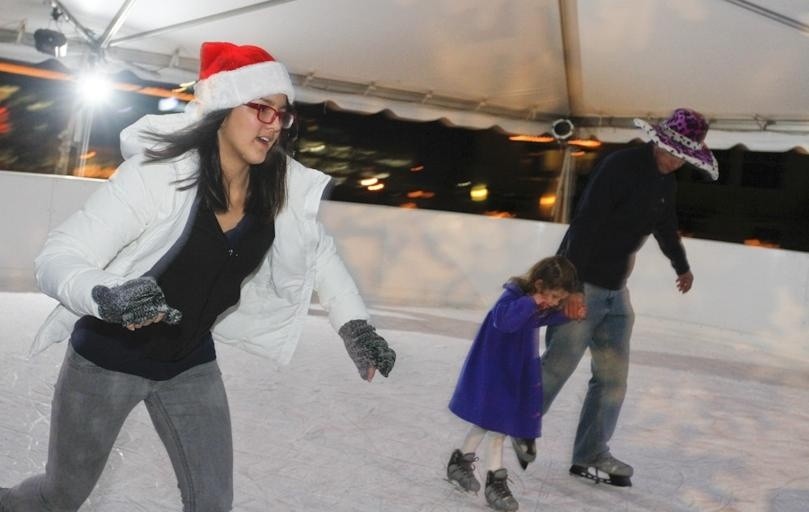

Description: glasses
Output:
[242,102,294,129]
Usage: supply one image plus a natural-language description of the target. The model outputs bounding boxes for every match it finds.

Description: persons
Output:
[0,42,395,512]
[447,255,586,512]
[508,108,719,478]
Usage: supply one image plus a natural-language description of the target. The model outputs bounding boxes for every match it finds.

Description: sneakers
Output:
[446,431,635,511]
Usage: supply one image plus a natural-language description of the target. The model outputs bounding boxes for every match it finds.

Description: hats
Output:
[633,108,719,180]
[194,42,294,116]
